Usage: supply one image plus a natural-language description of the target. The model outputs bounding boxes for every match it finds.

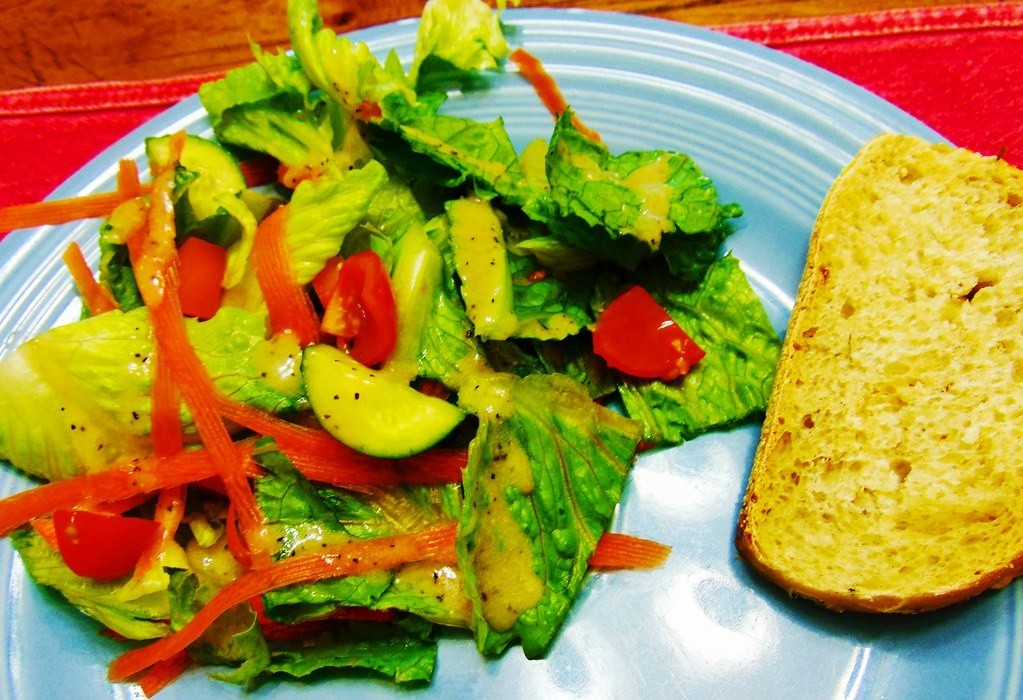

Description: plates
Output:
[0,11,1021,700]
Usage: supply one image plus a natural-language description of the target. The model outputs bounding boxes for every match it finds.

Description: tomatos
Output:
[52,507,158,579]
[322,252,399,366]
[594,284,705,380]
[176,238,226,320]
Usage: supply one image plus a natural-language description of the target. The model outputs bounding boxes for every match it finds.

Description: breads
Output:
[733,132,1023,613]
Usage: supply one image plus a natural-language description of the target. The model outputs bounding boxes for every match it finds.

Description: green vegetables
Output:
[8,0,779,689]
